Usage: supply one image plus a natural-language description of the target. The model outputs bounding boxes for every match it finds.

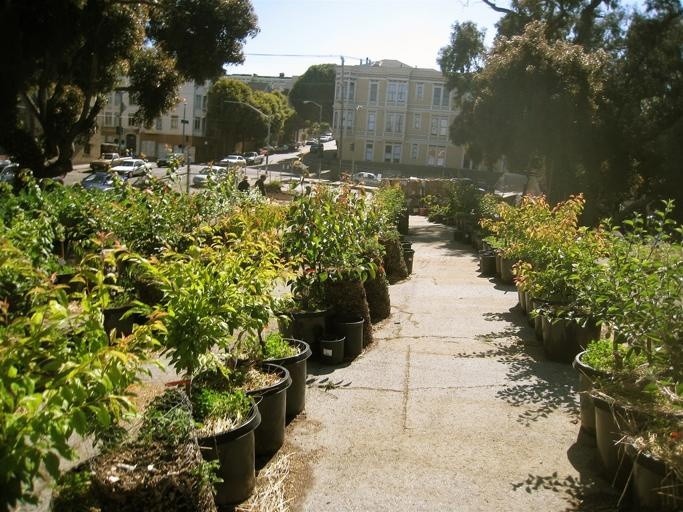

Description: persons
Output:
[237,175,249,195]
[254,175,266,196]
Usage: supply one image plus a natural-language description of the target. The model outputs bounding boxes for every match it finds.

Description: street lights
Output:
[302,99,323,186]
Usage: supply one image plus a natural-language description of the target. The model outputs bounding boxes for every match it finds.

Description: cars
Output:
[259,132,335,156]
[347,171,383,187]
[157,147,267,187]
[0,148,152,195]
